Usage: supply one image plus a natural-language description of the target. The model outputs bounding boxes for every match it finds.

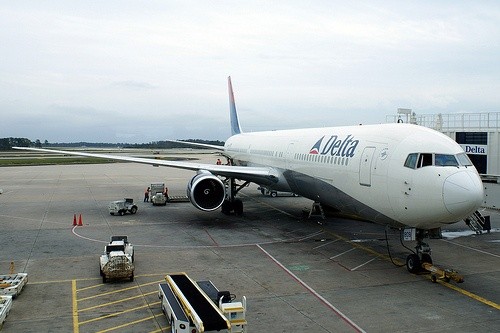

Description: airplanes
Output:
[13,75,487,274]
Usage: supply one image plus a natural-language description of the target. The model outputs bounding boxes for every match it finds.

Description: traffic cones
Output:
[77,213,83,225]
[72,213,78,225]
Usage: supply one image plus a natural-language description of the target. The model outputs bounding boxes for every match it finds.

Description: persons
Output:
[217,159,221,164]
[165,187,168,194]
[144,187,150,202]
